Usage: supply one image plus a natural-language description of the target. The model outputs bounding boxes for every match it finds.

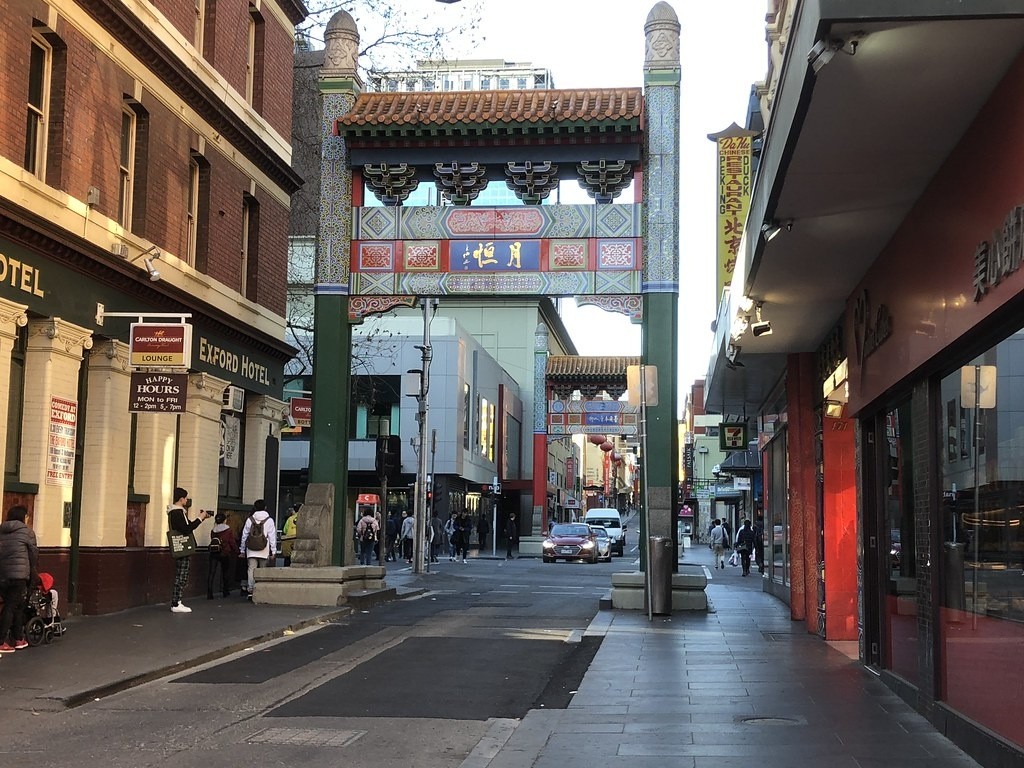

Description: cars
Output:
[542,522,599,565]
[573,525,614,562]
[773,526,790,550]
[891,530,902,567]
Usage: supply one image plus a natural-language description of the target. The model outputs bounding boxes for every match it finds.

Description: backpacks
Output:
[365,522,375,542]
[207,534,227,555]
[245,516,270,551]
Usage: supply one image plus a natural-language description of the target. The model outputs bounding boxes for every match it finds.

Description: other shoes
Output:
[506,555,514,559]
[715,565,718,568]
[720,560,724,569]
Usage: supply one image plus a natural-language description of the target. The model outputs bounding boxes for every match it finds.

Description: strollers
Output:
[24,566,65,648]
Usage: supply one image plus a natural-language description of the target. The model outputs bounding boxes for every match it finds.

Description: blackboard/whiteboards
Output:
[125,370,190,416]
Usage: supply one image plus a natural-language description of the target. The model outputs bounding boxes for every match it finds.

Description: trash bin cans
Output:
[644,536,674,615]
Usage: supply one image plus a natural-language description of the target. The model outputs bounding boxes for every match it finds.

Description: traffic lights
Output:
[434,483,443,504]
[426,492,432,500]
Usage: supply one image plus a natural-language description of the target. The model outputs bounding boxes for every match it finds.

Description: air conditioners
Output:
[221,385,245,413]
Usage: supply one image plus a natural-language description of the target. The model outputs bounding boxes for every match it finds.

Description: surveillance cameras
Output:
[285,416,296,429]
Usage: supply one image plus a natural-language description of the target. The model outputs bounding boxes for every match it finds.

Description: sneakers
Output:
[15,640,28,648]
[171,601,192,612]
[0,643,15,652]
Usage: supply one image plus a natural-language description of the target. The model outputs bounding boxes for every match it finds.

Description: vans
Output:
[585,508,628,558]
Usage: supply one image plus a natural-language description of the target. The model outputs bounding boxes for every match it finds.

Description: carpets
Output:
[262,636,605,721]
[166,623,389,683]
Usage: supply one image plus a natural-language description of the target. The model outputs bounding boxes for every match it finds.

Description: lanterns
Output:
[590,434,621,466]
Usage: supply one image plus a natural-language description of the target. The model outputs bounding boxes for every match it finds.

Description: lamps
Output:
[725,295,772,371]
[805,38,859,77]
[762,220,793,242]
[129,246,161,282]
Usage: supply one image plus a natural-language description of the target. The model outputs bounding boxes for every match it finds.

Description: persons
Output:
[385,510,397,562]
[0,506,39,654]
[357,510,379,566]
[444,511,457,562]
[506,513,517,559]
[432,512,443,562]
[283,502,305,567]
[711,519,729,569]
[37,573,66,634]
[211,513,244,599]
[549,518,557,532]
[722,518,731,538]
[709,521,716,549]
[476,514,490,549]
[240,499,277,600]
[453,509,473,563]
[401,511,414,564]
[738,520,754,577]
[167,488,211,613]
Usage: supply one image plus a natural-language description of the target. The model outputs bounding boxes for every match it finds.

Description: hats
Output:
[510,513,515,517]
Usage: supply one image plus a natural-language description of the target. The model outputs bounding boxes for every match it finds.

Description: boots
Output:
[222,587,230,597]
[206,590,214,600]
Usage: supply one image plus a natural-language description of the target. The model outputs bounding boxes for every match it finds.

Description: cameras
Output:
[200,510,214,518]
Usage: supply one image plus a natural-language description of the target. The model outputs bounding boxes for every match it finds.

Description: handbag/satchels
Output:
[450,530,460,545]
[722,528,730,549]
[737,543,748,554]
[728,550,738,567]
[167,512,195,558]
[443,520,451,535]
